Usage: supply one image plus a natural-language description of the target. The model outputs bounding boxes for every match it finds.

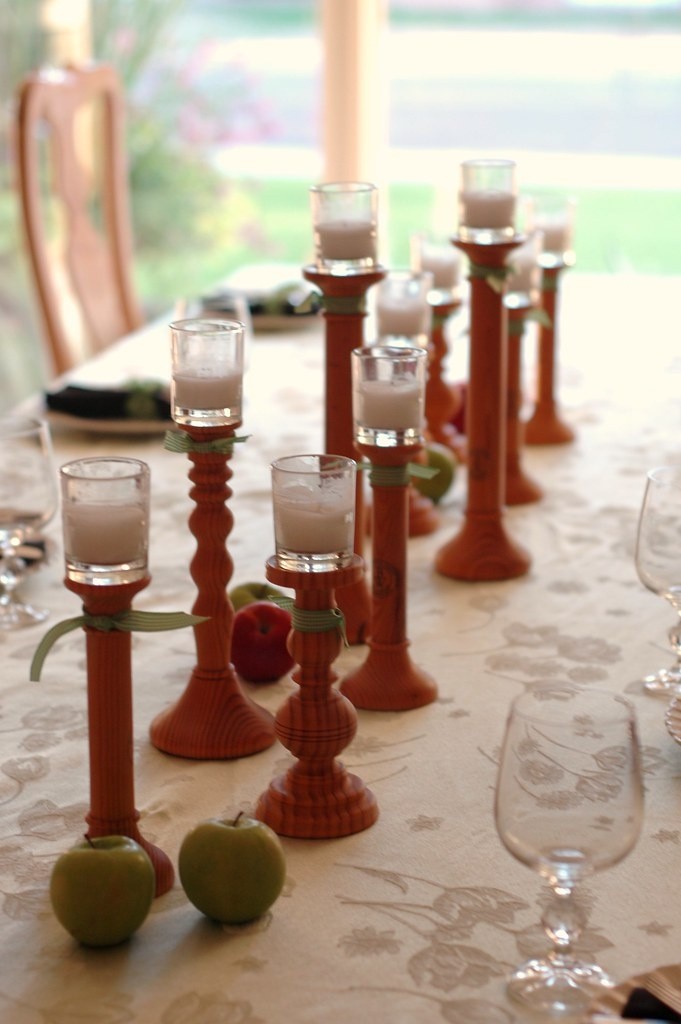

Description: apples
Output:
[179,818,286,924]
[230,582,295,683]
[50,837,156,945]
[417,447,455,502]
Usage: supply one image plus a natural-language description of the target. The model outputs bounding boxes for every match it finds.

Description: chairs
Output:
[14,55,149,381]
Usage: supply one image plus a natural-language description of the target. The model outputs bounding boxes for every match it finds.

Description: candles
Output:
[316,217,376,257]
[507,255,533,288]
[172,361,242,410]
[411,247,461,288]
[351,374,426,432]
[63,500,145,566]
[272,483,354,553]
[460,188,515,225]
[375,293,429,334]
[540,219,569,252]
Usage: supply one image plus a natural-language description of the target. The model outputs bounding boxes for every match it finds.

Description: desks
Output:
[0,261,681,1024]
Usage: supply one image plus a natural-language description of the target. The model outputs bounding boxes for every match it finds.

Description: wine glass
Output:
[0,414,59,631]
[634,464,681,693]
[492,683,644,1015]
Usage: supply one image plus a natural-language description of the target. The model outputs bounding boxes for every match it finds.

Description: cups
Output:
[412,193,575,307]
[309,181,379,276]
[457,159,517,243]
[270,454,357,571]
[350,345,429,448]
[60,456,151,587]
[168,318,246,427]
[372,268,433,354]
